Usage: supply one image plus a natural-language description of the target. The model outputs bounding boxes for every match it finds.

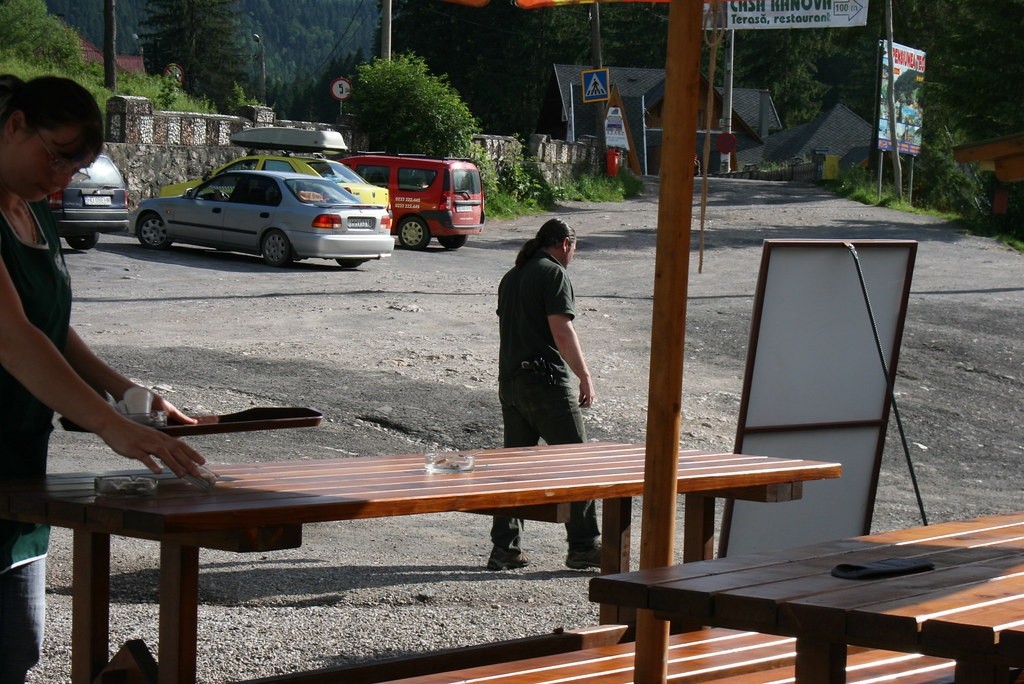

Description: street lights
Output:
[253,32,267,103]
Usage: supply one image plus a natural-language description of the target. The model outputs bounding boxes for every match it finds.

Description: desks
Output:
[587,512,1024,684]
[0,443,845,684]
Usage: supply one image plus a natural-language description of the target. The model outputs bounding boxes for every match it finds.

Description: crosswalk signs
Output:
[582,68,611,104]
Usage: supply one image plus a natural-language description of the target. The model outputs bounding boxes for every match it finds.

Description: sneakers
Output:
[487,547,528,570]
[566,542,603,569]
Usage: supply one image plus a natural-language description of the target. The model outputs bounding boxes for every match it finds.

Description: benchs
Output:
[401,627,957,684]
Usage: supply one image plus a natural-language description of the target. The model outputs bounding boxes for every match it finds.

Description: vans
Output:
[330,151,486,251]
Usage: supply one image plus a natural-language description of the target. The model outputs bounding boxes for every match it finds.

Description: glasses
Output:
[25,121,81,182]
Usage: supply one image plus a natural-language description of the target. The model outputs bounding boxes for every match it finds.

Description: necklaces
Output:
[1,199,37,245]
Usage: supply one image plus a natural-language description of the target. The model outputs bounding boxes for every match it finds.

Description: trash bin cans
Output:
[606,146,620,178]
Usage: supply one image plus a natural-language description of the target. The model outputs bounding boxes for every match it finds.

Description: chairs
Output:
[369,172,386,184]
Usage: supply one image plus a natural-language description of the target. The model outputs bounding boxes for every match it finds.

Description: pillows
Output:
[297,190,323,203]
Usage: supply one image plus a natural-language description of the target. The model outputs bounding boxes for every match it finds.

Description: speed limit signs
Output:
[330,78,353,102]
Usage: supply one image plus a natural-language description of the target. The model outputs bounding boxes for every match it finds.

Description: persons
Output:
[0,75,205,684]
[214,184,230,201]
[486,215,602,571]
[693,155,700,175]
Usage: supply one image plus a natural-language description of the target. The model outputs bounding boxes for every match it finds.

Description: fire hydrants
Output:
[607,147,622,178]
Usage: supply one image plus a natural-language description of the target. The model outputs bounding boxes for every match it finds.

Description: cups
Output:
[124,388,154,413]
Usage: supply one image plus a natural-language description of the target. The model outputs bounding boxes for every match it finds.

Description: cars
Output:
[128,169,396,268]
[49,154,131,252]
[157,127,393,238]
